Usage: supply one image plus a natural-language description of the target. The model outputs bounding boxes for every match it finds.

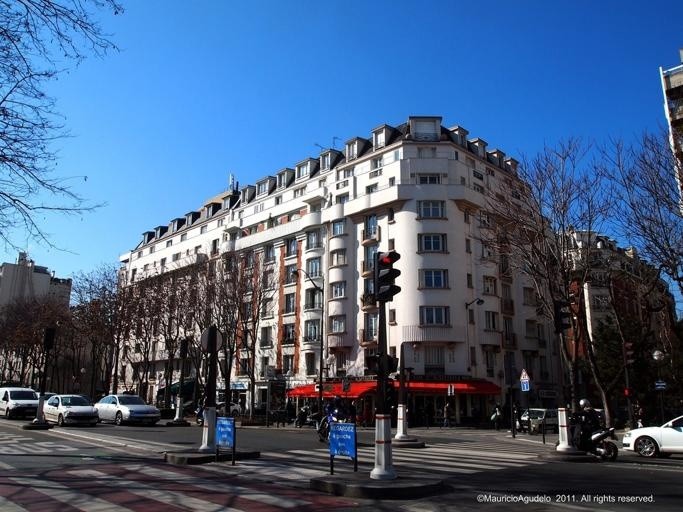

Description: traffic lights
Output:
[554,299,573,332]
[622,341,636,367]
[373,250,402,302]
[623,387,632,397]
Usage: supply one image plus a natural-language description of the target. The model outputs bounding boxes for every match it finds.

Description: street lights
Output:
[289,266,324,422]
[653,350,667,425]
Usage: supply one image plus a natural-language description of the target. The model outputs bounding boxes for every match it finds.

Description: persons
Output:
[568,398,605,454]
[442,402,452,429]
[292,399,358,440]
[491,400,505,431]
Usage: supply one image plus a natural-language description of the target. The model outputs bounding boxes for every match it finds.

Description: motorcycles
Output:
[315,410,352,442]
[566,402,618,461]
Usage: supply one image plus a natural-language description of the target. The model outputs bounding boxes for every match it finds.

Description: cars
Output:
[214,402,242,416]
[0,385,161,428]
[244,402,267,414]
[622,414,682,460]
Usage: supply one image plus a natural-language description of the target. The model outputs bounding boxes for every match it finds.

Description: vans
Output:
[515,408,557,435]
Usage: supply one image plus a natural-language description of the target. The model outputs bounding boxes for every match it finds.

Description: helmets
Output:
[579,399,589,408]
